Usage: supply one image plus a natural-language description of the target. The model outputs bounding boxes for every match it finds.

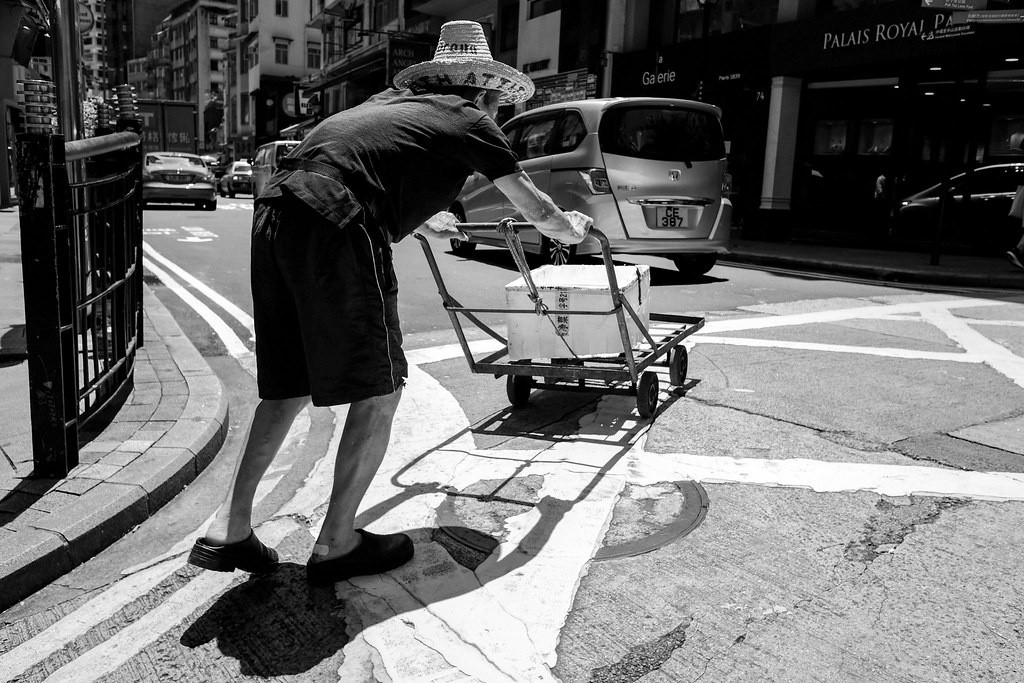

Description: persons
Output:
[188,21,594,587]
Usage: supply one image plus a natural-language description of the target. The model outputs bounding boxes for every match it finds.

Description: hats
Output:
[392,20,536,106]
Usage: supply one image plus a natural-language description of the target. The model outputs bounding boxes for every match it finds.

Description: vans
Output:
[446,95,733,276]
[253,140,301,198]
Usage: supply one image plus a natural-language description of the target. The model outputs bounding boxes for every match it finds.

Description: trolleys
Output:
[408,221,705,418]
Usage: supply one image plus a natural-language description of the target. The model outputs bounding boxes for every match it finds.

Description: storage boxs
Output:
[506,263,651,359]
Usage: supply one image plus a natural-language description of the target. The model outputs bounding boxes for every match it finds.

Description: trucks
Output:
[135,98,201,155]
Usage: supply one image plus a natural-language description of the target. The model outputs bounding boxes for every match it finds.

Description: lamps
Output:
[599,48,616,66]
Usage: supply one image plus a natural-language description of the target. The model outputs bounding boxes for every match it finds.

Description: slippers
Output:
[186,527,279,572]
[305,528,415,583]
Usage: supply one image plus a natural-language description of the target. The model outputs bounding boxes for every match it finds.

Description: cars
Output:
[218,157,253,198]
[891,162,1024,242]
[141,151,217,210]
